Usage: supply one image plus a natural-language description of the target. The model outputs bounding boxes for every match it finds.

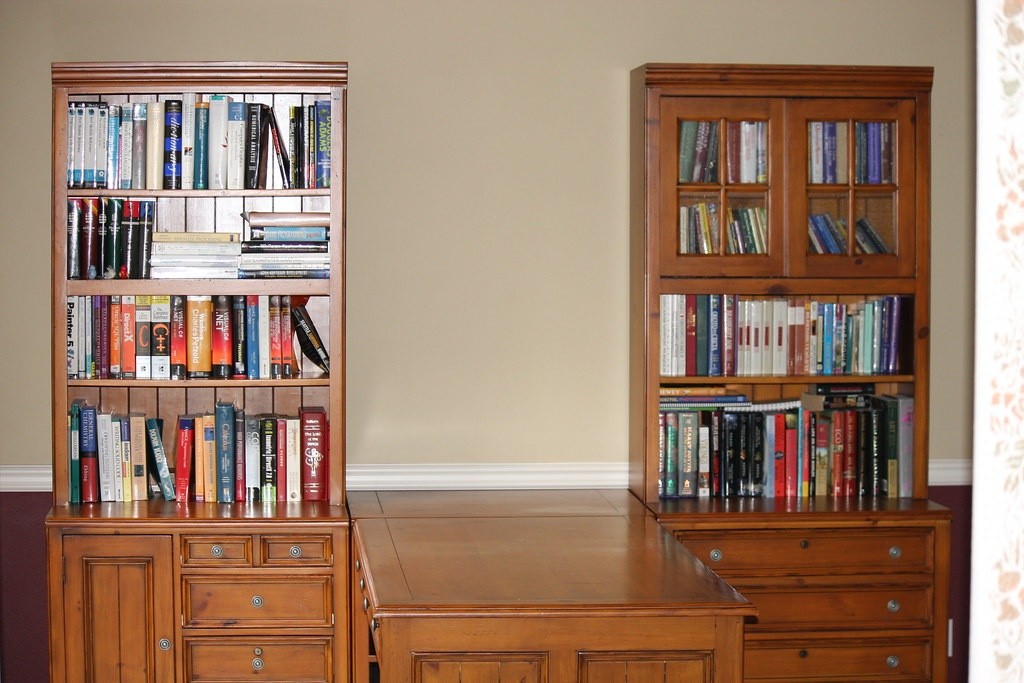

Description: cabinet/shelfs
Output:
[654,498,950,682]
[49,62,348,512]
[629,60,936,508]
[42,501,354,682]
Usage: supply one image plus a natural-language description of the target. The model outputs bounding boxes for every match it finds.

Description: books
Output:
[657,119,912,502]
[68,88,328,505]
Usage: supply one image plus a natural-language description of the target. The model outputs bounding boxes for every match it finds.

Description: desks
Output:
[344,488,763,683]
